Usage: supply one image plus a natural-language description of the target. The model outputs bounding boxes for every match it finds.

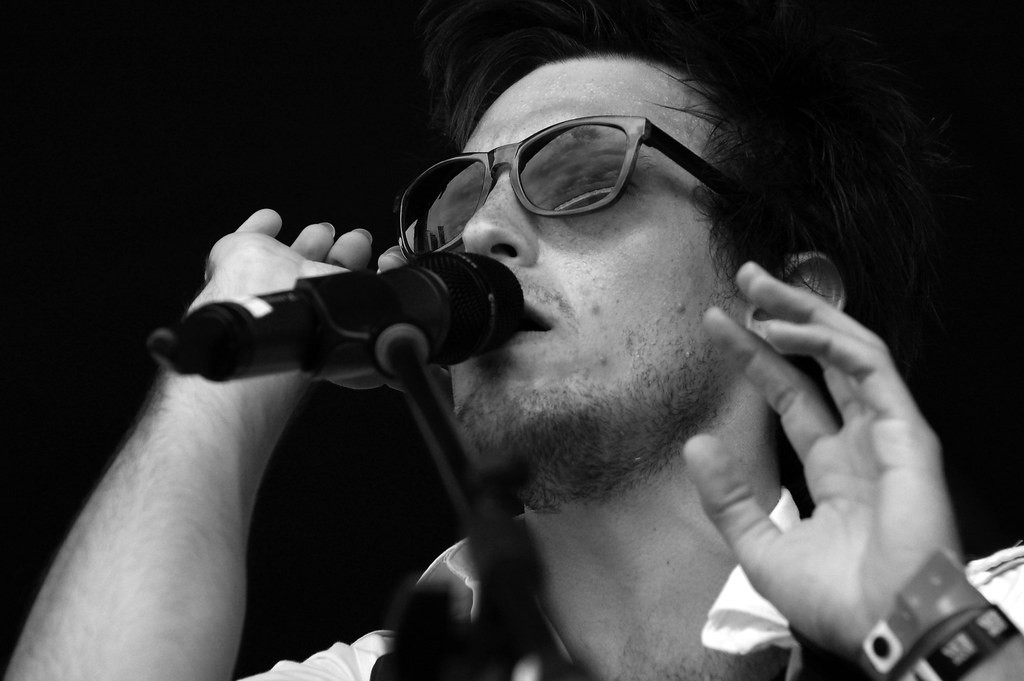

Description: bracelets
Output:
[860,550,1020,681]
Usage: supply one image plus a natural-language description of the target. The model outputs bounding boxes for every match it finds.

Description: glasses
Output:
[394,115,755,261]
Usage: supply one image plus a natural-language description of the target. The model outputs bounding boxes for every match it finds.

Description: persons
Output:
[0,0,1024,681]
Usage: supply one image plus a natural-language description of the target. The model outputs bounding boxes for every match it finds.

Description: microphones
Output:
[151,251,526,384]
